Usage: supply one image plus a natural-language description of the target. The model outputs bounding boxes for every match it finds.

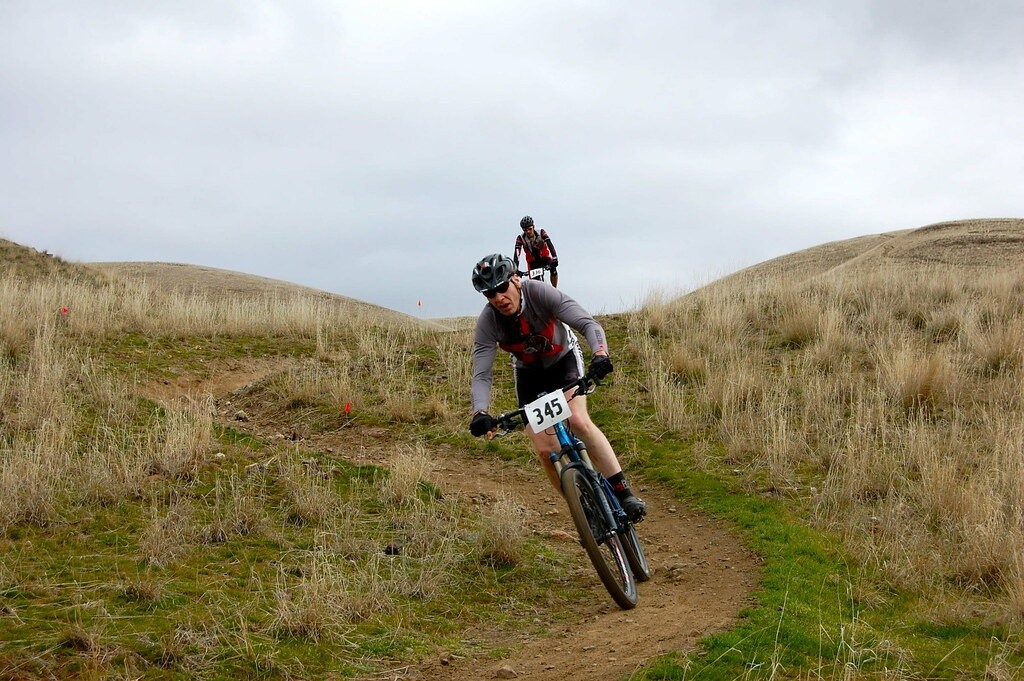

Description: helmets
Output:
[471,252,518,293]
[520,215,534,228]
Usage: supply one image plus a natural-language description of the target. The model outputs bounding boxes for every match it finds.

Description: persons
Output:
[469,253,647,548]
[514,216,559,288]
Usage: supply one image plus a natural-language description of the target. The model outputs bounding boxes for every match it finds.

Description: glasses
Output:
[482,276,514,299]
[523,227,533,232]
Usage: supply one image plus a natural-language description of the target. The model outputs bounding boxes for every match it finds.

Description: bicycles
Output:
[516,258,557,283]
[466,356,651,611]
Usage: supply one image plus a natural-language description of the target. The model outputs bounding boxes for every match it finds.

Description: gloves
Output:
[515,269,523,279]
[588,355,614,386]
[550,259,558,268]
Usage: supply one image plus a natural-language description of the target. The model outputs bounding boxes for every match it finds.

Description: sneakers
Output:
[621,495,644,521]
[584,509,600,540]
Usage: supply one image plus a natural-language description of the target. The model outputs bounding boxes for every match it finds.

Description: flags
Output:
[345,401,350,413]
